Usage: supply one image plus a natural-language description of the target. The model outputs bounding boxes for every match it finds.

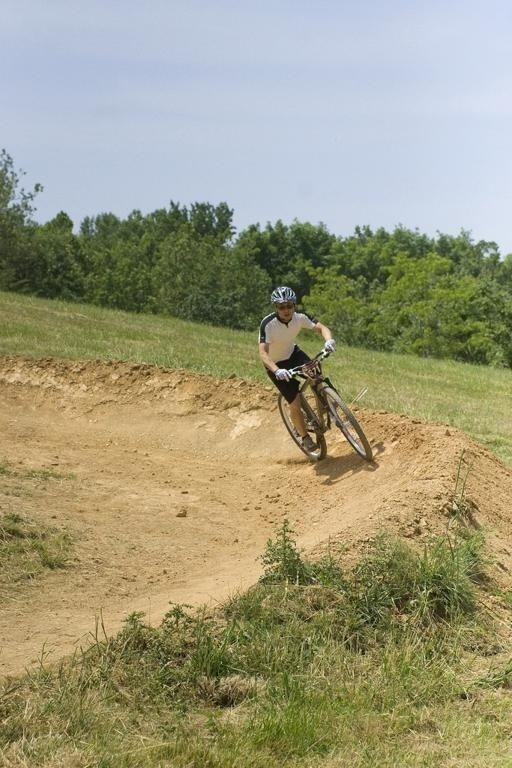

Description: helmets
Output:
[271,286,297,303]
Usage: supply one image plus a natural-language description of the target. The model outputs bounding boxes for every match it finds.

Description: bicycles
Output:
[275,345,373,465]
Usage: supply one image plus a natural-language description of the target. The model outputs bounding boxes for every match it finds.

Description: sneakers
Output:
[302,435,317,452]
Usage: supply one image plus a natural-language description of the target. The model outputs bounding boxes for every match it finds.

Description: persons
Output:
[257,285,336,451]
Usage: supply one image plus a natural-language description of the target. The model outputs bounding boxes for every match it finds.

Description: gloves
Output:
[274,368,293,382]
[325,339,337,353]
